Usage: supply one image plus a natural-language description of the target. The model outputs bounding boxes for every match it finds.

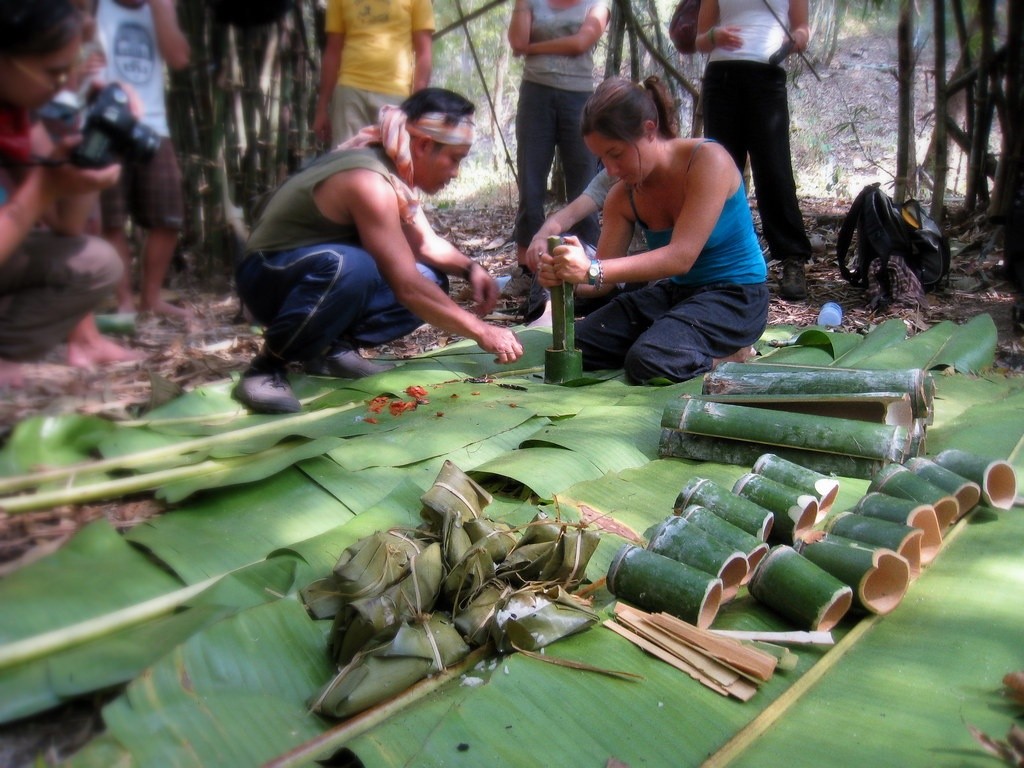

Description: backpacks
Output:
[838,182,953,287]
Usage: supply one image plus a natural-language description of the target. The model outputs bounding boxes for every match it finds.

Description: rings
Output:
[537,266,541,273]
[538,252,543,257]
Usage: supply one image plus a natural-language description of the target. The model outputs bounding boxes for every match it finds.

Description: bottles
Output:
[815,298,843,328]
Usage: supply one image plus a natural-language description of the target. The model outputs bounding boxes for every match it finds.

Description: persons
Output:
[231,89,525,414]
[0,0,811,415]
[536,74,769,388]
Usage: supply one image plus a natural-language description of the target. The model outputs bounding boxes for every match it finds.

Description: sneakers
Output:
[501,266,533,297]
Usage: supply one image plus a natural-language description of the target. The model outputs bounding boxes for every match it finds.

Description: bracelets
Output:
[709,27,716,46]
[596,259,604,292]
[465,261,475,280]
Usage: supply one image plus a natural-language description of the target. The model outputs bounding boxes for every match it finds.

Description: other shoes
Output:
[321,351,395,378]
[236,375,301,412]
[782,265,807,301]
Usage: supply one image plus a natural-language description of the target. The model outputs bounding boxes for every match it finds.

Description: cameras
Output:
[72,83,160,170]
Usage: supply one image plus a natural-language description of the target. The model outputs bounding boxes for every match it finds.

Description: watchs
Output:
[588,260,599,285]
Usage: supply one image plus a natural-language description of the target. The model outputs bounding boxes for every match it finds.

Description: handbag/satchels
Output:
[669,0,701,55]
